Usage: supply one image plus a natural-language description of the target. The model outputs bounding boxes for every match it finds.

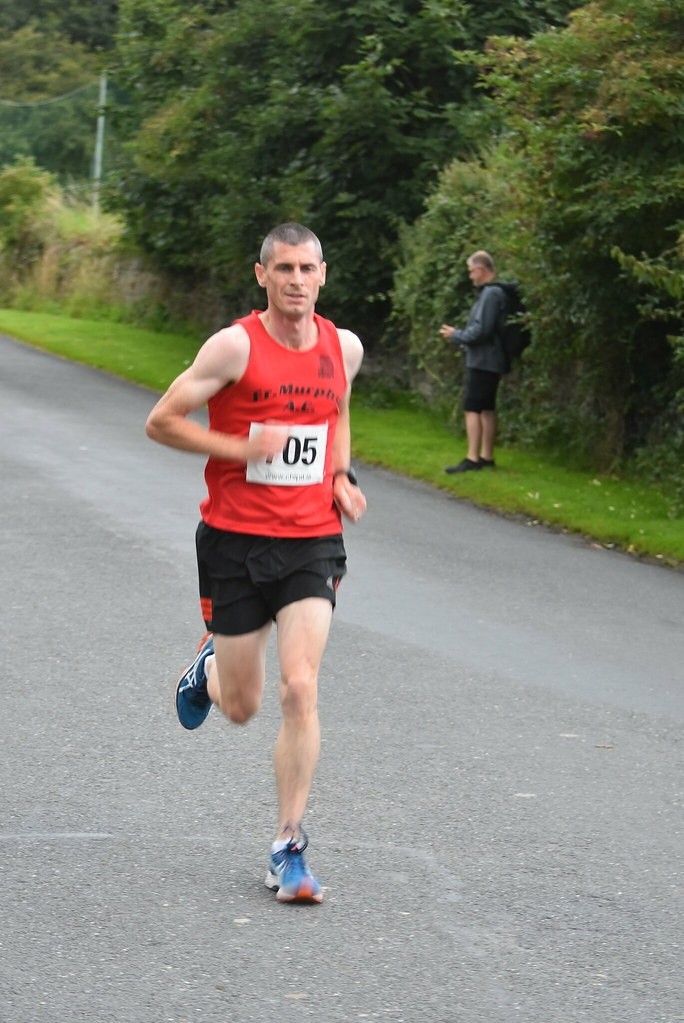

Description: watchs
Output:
[334,467,360,485]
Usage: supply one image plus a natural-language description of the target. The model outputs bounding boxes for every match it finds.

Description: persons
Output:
[440,250,508,475]
[145,221,364,906]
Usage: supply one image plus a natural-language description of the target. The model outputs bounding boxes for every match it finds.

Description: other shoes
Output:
[444,456,496,475]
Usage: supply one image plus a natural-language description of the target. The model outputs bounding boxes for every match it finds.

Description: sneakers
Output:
[172,628,215,730]
[265,835,325,904]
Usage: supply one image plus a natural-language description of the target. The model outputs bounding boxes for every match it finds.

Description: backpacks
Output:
[482,280,532,364]
